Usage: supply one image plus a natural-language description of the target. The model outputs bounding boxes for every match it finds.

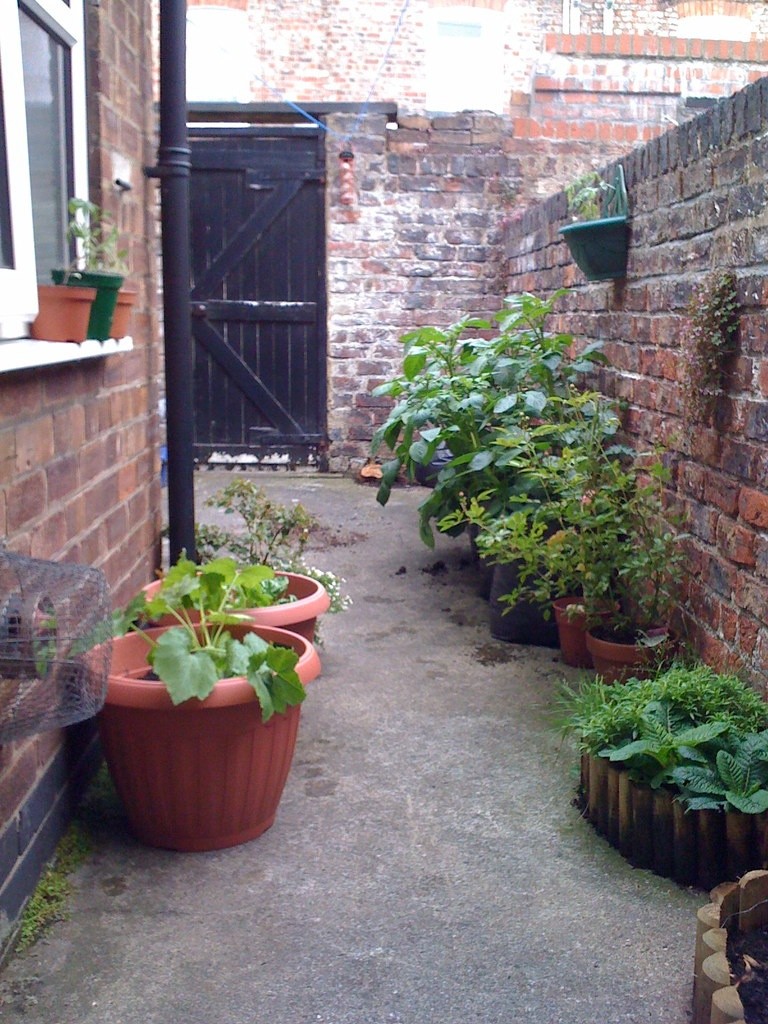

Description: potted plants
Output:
[129,549,330,646]
[34,270,96,344]
[82,561,319,849]
[371,293,673,686]
[560,165,631,283]
[50,196,124,341]
